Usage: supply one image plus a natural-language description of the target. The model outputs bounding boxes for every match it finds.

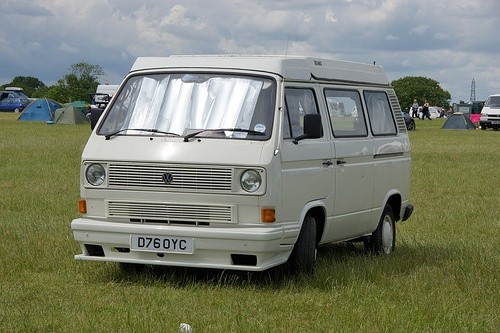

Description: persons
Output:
[437,104,453,119]
[412,99,421,120]
[421,99,432,120]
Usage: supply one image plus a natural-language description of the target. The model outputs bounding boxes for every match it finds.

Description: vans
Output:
[409,106,440,120]
[479,94,500,131]
[68,52,415,274]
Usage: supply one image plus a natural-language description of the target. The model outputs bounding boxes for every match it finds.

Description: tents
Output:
[55,101,89,125]
[442,112,476,130]
[18,98,63,122]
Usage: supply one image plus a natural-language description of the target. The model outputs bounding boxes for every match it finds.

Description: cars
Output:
[0,89,34,112]
